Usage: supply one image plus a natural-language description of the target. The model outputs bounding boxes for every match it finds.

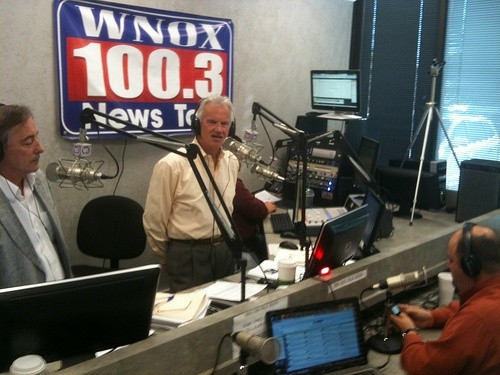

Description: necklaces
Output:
[6,175,46,229]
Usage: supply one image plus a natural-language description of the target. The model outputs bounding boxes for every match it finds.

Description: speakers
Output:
[455,158,500,223]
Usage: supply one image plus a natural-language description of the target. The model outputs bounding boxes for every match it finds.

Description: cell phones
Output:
[389,304,402,316]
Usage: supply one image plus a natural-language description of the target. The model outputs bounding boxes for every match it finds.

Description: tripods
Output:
[400,66,459,226]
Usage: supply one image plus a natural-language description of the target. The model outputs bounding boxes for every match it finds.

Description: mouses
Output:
[279,241,298,249]
[280,231,296,239]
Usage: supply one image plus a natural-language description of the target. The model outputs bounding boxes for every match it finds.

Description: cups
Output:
[438,271,456,306]
[9,355,46,375]
[278,257,297,287]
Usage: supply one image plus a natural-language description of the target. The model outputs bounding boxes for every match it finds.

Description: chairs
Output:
[70,196,147,277]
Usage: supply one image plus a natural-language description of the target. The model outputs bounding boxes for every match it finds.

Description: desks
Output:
[365,286,458,375]
[150,191,459,335]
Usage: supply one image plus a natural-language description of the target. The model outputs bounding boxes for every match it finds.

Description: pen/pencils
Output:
[154,296,174,306]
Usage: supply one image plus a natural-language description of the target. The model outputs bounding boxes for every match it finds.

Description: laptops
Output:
[266,296,386,375]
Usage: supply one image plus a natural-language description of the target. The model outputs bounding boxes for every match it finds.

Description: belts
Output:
[171,237,224,244]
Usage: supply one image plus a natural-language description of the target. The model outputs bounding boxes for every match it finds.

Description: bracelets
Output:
[402,328,418,336]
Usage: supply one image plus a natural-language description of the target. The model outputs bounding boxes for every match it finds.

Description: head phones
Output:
[458,223,480,278]
[191,97,236,136]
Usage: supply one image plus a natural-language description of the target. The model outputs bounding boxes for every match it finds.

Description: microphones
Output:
[45,162,109,182]
[366,271,421,291]
[222,136,264,164]
[250,164,287,183]
[229,330,282,364]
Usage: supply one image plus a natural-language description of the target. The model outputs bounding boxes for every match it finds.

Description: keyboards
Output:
[271,212,295,233]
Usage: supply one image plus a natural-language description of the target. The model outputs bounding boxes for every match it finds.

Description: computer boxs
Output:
[391,158,447,211]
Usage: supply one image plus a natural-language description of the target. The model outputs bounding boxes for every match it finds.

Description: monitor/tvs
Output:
[303,135,385,281]
[0,263,161,375]
[379,165,442,218]
[311,69,360,115]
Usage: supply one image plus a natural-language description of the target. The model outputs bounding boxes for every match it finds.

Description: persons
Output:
[142,95,240,293]
[226,134,277,273]
[390,223,500,375]
[0,103,73,289]
